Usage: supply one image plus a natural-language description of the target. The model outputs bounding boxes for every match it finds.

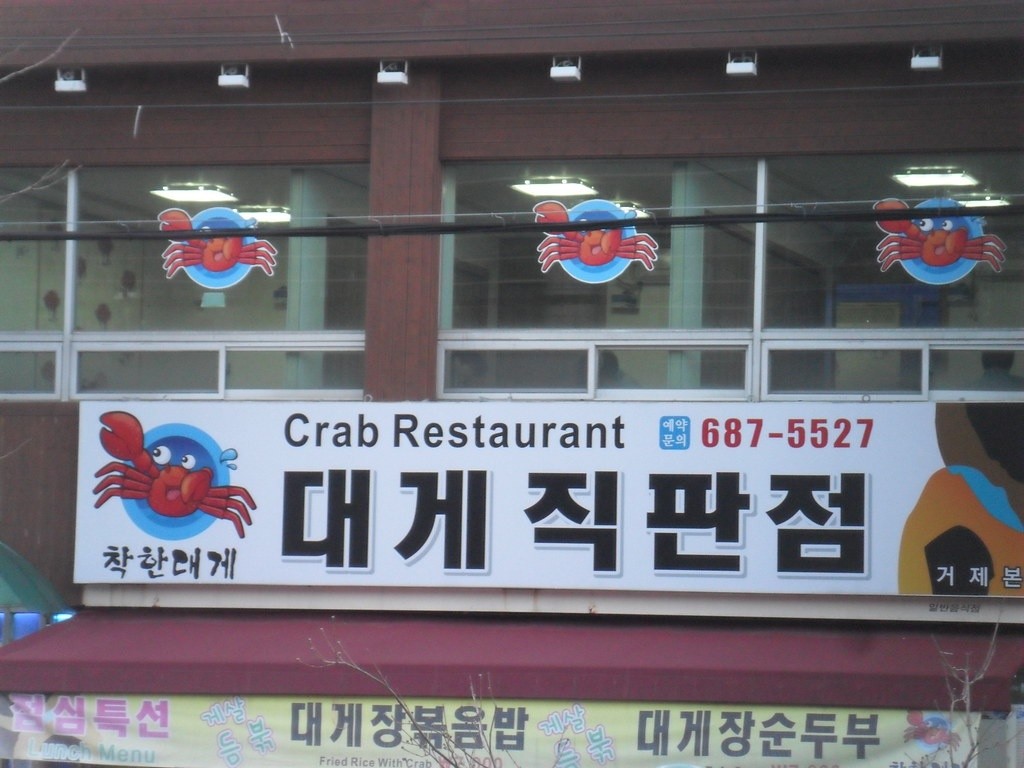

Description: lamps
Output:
[549,56,582,82]
[886,166,983,190]
[53,66,87,92]
[611,187,652,217]
[233,186,292,222]
[725,49,758,76]
[507,163,600,197]
[911,43,943,71]
[149,166,238,201]
[217,62,249,89]
[949,194,1012,207]
[376,59,409,85]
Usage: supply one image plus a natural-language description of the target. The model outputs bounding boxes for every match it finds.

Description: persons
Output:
[974,350,1024,391]
[598,350,637,389]
